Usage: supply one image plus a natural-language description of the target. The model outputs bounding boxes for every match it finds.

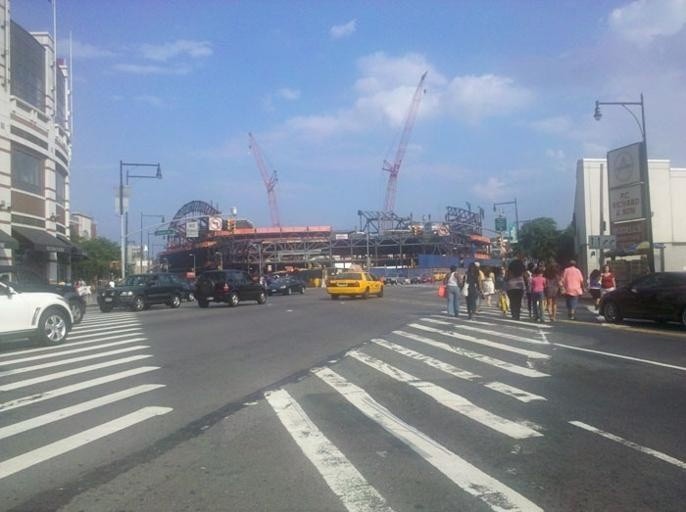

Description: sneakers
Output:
[567,309,577,320]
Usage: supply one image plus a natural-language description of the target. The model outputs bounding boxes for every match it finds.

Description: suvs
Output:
[192,270,267,308]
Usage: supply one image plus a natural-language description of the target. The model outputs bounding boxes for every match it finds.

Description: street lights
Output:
[119,159,164,278]
[592,91,657,273]
[493,196,521,246]
[139,213,166,274]
[187,253,196,273]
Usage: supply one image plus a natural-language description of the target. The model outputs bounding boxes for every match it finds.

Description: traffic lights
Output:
[227,219,230,230]
[417,225,423,235]
[497,237,501,247]
[412,225,416,237]
[231,220,236,230]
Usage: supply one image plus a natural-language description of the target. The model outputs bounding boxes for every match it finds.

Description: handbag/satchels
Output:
[439,286,447,297]
[461,283,469,297]
[498,291,509,313]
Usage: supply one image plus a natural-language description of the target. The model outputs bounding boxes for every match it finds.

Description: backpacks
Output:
[601,274,615,289]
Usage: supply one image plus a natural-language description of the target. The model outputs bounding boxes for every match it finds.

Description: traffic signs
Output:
[155,229,176,236]
[495,218,506,230]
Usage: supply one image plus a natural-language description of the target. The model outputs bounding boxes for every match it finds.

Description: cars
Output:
[97,273,187,313]
[268,277,307,296]
[177,278,197,302]
[598,270,686,326]
[0,264,87,323]
[0,279,74,346]
[383,270,450,286]
[326,263,385,298]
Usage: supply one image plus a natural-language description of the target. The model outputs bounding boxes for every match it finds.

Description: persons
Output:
[59,276,111,296]
[441,259,616,322]
